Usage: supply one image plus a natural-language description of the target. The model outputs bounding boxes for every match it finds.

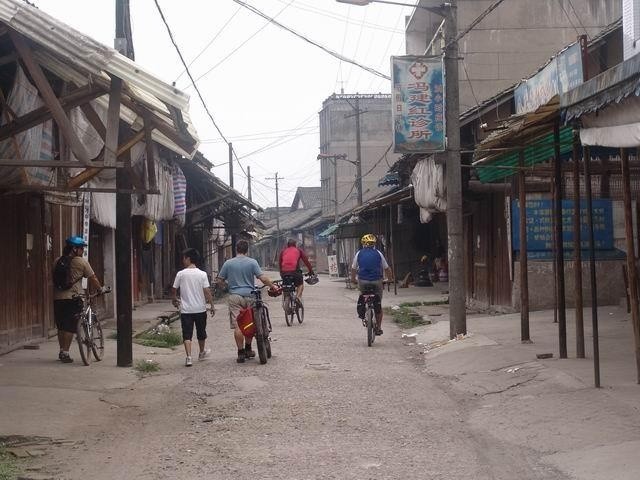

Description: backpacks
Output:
[54,255,85,290]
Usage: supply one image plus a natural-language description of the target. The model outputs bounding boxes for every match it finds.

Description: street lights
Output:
[336,0,466,334]
[317,153,362,217]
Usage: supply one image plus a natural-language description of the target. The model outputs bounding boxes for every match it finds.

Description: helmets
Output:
[305,278,319,285]
[267,286,283,297]
[66,236,87,246]
[361,234,377,245]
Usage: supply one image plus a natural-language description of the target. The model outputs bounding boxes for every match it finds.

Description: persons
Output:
[351,234,392,335]
[279,239,317,313]
[171,248,216,367]
[216,240,277,363]
[52,237,109,363]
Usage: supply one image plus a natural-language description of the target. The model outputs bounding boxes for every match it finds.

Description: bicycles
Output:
[280,271,315,326]
[75,286,112,366]
[252,279,286,364]
[351,279,394,346]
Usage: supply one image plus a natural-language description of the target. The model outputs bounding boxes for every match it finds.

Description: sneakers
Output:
[62,352,74,363]
[246,350,255,358]
[198,349,212,360]
[184,356,192,365]
[237,354,245,362]
[59,352,63,359]
